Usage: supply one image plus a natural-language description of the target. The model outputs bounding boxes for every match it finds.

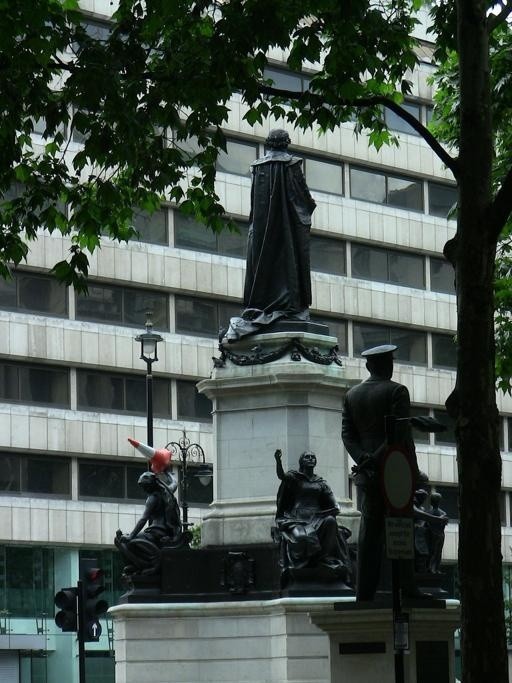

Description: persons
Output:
[244,129,317,321]
[274,449,344,570]
[114,472,182,577]
[341,344,446,608]
[413,489,448,575]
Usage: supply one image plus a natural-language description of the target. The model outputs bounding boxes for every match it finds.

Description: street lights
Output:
[158,428,213,546]
[133,310,165,539]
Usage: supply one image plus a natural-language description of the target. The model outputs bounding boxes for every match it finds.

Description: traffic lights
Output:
[53,557,110,643]
